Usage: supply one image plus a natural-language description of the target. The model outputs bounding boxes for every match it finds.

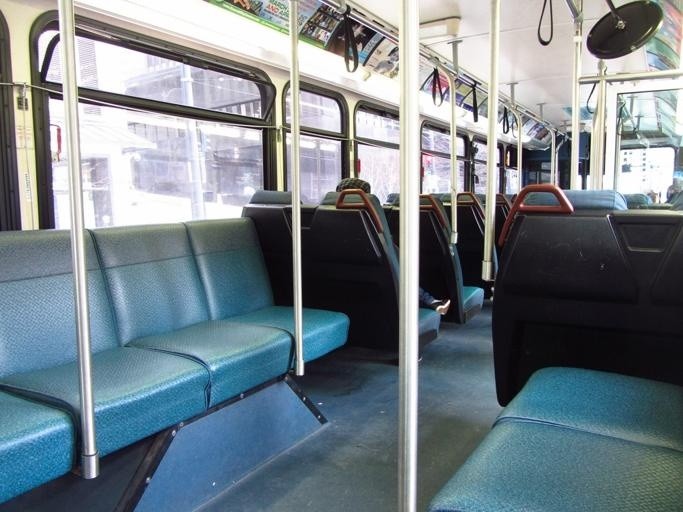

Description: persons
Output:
[336,177,451,315]
[372,47,399,75]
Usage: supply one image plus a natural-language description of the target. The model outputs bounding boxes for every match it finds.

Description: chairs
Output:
[429,424,683,512]
[490,371,683,458]
[87,227,292,413]
[2,392,80,504]
[487,182,682,403]
[2,230,203,472]
[190,217,349,376]
[246,179,486,346]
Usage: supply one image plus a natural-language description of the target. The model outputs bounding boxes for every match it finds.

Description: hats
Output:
[336,178,370,193]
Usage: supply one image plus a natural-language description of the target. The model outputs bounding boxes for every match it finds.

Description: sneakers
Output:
[430,297,451,315]
